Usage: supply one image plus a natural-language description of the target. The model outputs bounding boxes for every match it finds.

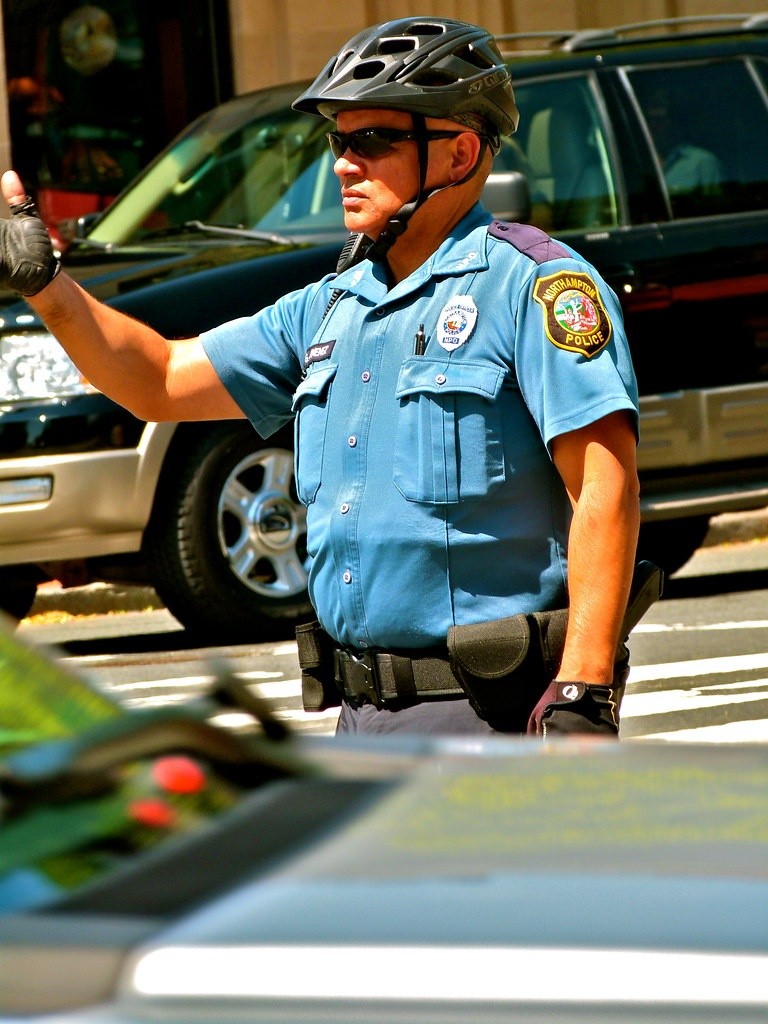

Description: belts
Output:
[334,647,464,712]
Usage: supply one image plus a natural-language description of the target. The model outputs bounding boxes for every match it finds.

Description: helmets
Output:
[290,18,519,136]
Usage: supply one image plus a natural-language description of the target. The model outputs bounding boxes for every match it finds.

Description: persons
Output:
[648,84,721,194]
[0,16,642,741]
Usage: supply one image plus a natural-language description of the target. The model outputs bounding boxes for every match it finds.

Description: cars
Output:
[0,606,768,1023]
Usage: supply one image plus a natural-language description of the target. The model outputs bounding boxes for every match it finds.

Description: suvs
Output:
[1,8,767,648]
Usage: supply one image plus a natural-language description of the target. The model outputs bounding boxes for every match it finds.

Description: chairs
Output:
[526,108,603,232]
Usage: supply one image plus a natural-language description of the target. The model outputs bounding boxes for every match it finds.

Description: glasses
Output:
[326,126,496,159]
